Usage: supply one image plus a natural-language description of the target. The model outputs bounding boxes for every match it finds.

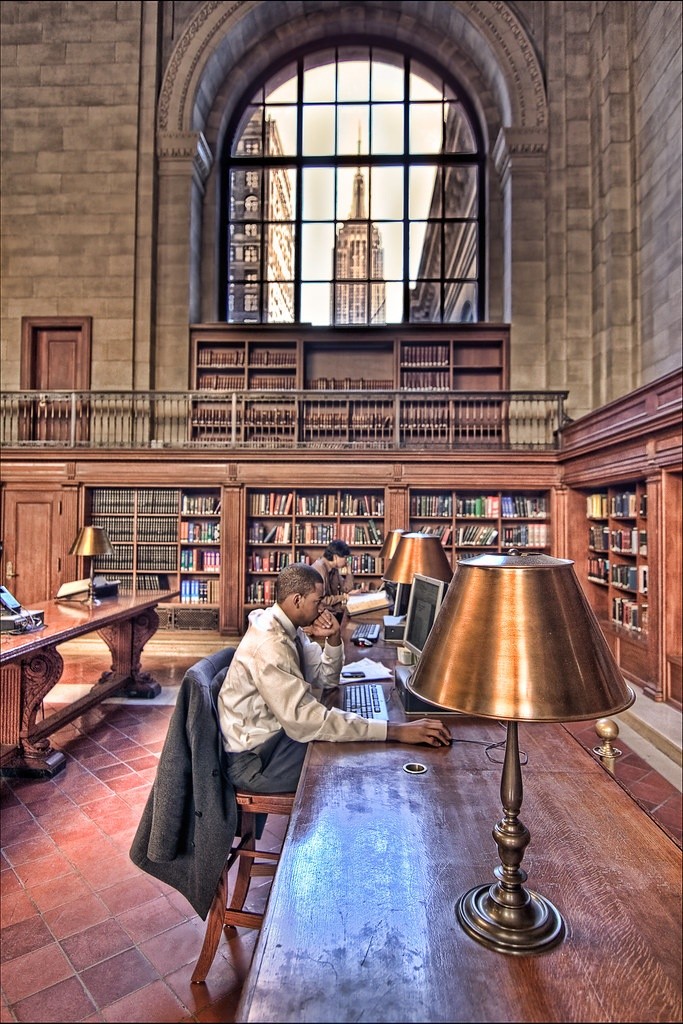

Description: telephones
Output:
[0,586,21,610]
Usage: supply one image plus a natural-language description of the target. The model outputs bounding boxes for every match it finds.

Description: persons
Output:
[217,563,451,794]
[310,538,355,626]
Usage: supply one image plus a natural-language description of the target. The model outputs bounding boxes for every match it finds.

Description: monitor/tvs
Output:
[391,573,451,674]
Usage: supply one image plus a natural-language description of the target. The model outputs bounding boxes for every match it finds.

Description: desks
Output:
[0,588,180,781]
[235,608,683,1023]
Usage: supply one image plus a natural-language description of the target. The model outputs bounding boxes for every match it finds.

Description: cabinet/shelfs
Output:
[189,330,511,451]
[78,482,225,636]
[241,483,389,638]
[406,484,559,573]
[585,482,648,688]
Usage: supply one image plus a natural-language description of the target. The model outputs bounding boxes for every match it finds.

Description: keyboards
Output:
[344,684,390,722]
[350,623,381,643]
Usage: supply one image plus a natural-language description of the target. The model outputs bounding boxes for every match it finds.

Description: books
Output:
[418,524,547,548]
[248,550,382,574]
[346,591,390,613]
[353,580,372,592]
[248,490,385,517]
[458,553,477,561]
[94,546,220,573]
[249,521,383,545]
[412,495,547,519]
[196,348,297,424]
[308,376,393,391]
[585,491,650,635]
[91,489,221,515]
[96,574,219,603]
[306,411,382,428]
[400,344,449,427]
[92,517,220,543]
[246,579,278,607]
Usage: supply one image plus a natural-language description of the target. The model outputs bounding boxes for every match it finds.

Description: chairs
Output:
[129,647,297,984]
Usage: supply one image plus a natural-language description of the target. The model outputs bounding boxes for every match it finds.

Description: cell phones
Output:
[342,671,366,679]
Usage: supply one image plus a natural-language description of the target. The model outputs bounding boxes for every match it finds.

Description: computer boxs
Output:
[394,664,462,715]
[384,615,407,642]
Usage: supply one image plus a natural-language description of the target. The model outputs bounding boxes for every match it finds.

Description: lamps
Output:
[380,529,455,665]
[68,524,115,606]
[378,528,408,560]
[405,548,636,957]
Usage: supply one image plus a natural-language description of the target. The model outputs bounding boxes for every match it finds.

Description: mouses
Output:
[353,637,373,648]
[417,729,452,747]
